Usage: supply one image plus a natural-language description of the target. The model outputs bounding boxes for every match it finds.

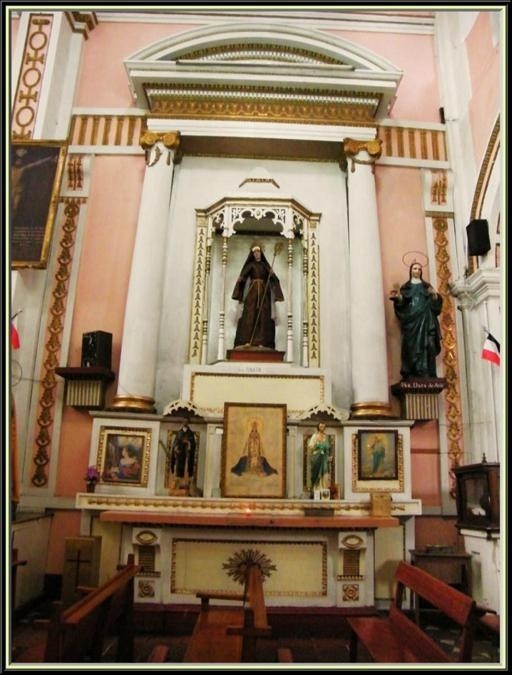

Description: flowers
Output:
[83,465,100,483]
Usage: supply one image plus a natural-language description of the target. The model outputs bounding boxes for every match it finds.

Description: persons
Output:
[390,263,442,378]
[309,419,334,499]
[231,242,285,352]
[242,421,266,477]
[369,435,387,478]
[118,446,142,479]
[169,413,196,496]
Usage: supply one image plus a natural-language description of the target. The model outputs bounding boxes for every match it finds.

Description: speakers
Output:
[81,331,112,369]
[466,220,490,255]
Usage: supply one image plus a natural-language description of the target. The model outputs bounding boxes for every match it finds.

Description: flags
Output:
[479,334,500,367]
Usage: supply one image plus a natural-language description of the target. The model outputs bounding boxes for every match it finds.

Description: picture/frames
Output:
[10,139,67,270]
[221,401,287,499]
[344,427,411,500]
[86,418,161,497]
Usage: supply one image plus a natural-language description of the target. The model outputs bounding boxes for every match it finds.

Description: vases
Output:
[86,484,96,493]
